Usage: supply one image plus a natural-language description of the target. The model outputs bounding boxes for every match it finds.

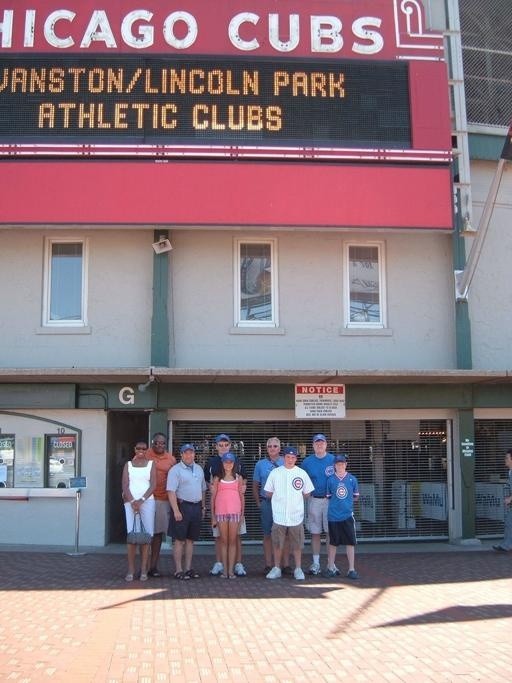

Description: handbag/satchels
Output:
[126,531,153,545]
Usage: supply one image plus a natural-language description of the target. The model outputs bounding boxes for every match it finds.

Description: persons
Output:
[121,440,157,581]
[492,449,512,551]
[210,453,245,579]
[166,443,208,580]
[252,437,294,575]
[204,434,247,576]
[326,456,360,579]
[299,434,341,575]
[263,447,315,580]
[132,433,176,576]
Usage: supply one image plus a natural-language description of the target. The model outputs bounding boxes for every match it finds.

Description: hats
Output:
[215,434,231,442]
[221,453,235,462]
[312,433,326,441]
[179,444,195,453]
[283,447,297,455]
[335,455,346,463]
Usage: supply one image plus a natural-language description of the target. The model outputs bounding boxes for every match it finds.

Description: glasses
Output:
[216,443,230,447]
[267,445,278,448]
[134,447,148,451]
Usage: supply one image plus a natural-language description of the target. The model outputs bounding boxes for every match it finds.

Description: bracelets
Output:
[141,496,146,502]
[202,506,207,510]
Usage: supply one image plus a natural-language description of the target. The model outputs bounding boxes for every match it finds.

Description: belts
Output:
[314,496,326,498]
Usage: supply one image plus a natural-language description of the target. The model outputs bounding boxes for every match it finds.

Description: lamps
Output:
[151,235,173,255]
[460,217,477,237]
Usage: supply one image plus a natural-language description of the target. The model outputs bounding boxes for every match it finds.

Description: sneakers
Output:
[125,562,359,581]
